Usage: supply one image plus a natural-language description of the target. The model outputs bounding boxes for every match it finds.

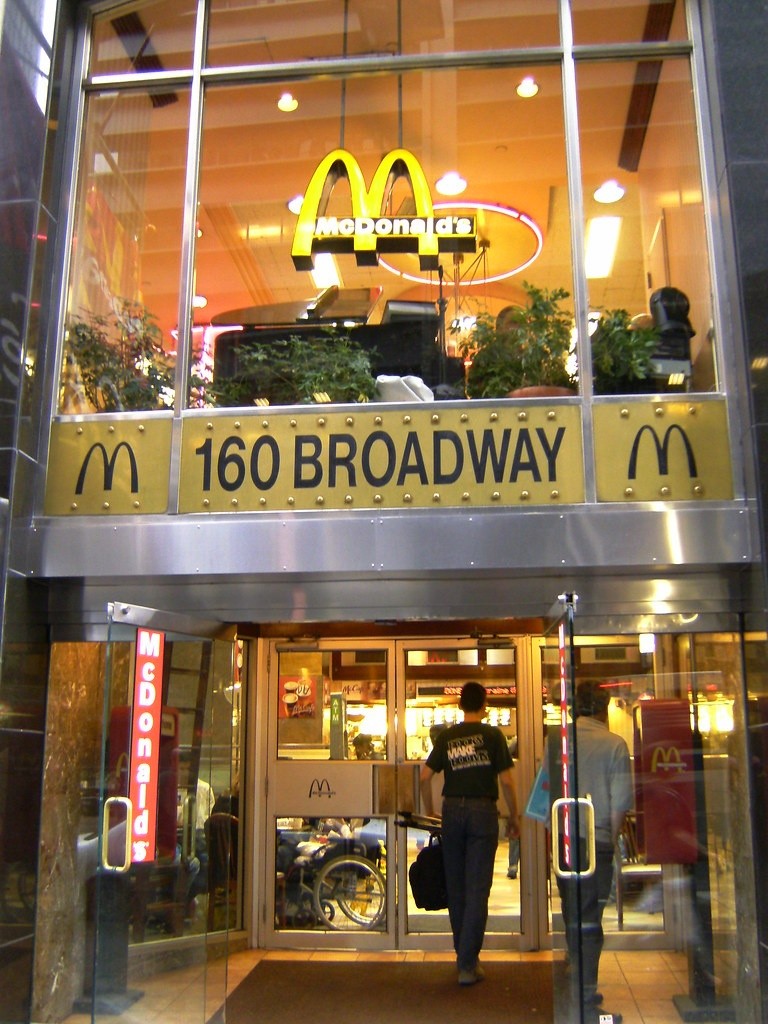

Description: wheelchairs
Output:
[285,837,389,933]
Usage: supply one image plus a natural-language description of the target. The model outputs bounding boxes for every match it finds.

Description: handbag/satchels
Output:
[409,833,448,911]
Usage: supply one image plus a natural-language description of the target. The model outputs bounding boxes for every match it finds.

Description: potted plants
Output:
[447,281,578,398]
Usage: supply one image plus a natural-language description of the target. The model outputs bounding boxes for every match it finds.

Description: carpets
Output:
[207,959,564,1024]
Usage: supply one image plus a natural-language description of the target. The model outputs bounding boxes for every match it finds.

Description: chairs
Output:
[130,861,187,942]
[204,813,288,932]
[613,811,661,932]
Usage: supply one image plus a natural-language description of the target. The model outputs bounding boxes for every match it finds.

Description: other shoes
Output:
[591,994,603,1005]
[287,900,311,918]
[458,966,485,986]
[507,871,516,879]
[569,1006,622,1024]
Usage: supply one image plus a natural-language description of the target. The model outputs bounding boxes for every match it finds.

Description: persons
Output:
[542,679,632,1024]
[177,681,547,986]
